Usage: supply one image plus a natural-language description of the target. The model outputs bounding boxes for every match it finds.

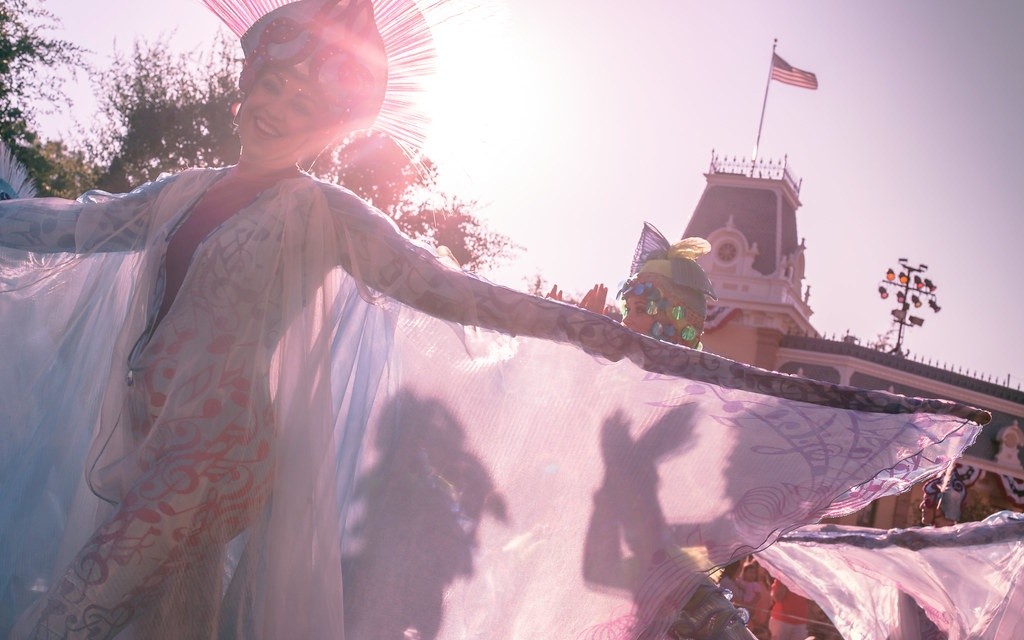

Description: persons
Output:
[0,0,1024,640]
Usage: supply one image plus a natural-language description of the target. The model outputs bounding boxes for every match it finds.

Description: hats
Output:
[202,0,437,147]
[922,465,968,522]
[616,221,718,350]
[0,142,37,201]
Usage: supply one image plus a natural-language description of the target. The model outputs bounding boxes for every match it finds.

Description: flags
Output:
[770,51,819,90]
